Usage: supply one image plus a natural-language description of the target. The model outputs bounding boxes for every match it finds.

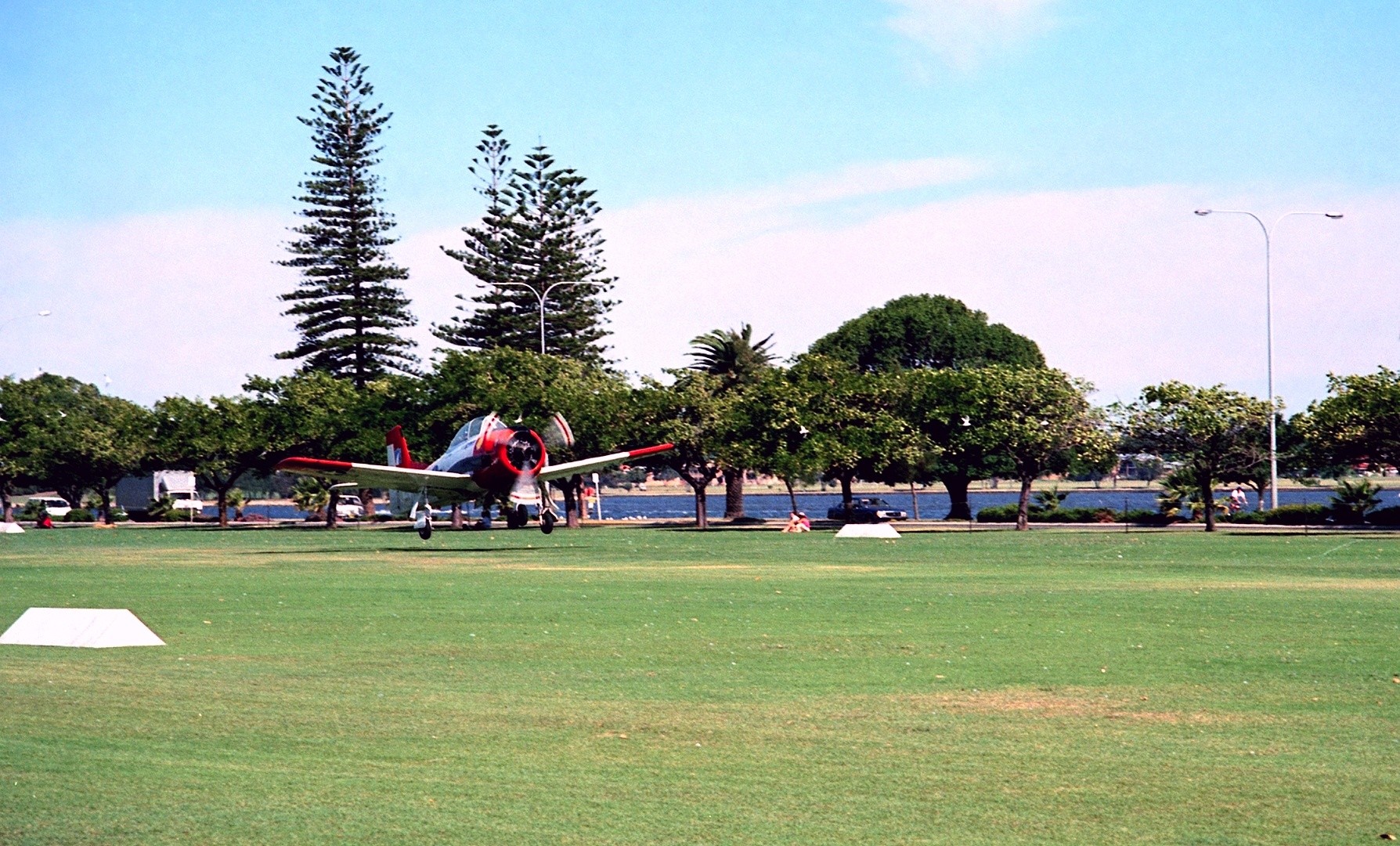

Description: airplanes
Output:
[274,406,677,540]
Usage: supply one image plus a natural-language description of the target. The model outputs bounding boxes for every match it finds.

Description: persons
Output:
[781,512,810,533]
[0,503,53,529]
[1230,486,1247,513]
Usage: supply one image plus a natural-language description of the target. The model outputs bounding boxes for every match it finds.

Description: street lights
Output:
[476,281,607,354]
[1195,207,1345,510]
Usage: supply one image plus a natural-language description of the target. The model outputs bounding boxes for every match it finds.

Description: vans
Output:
[160,490,204,514]
[29,497,72,516]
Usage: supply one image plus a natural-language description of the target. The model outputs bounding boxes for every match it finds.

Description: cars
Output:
[335,495,366,521]
[371,497,393,522]
[827,496,907,525]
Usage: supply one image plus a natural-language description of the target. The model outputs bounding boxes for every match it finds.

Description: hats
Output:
[799,512,805,516]
